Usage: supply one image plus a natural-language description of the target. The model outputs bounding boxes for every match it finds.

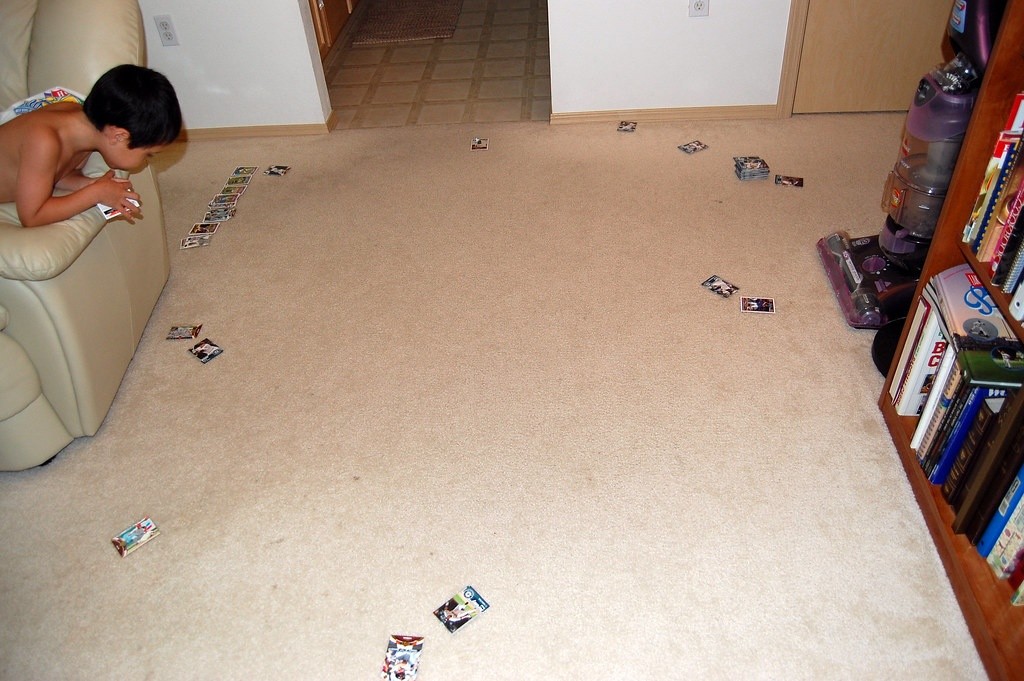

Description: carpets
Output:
[352,0,464,44]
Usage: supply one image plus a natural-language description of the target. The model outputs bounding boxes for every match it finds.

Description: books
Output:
[963,91,1024,328]
[889,265,1024,607]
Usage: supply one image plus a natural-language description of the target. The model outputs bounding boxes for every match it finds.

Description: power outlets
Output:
[689,0,709,17]
[153,13,181,46]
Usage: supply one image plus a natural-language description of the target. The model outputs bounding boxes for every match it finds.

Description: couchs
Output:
[0,0,172,472]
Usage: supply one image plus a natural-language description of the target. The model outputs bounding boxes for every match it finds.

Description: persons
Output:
[0,65,181,227]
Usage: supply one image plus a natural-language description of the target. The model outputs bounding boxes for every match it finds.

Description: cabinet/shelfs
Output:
[877,0,1024,681]
